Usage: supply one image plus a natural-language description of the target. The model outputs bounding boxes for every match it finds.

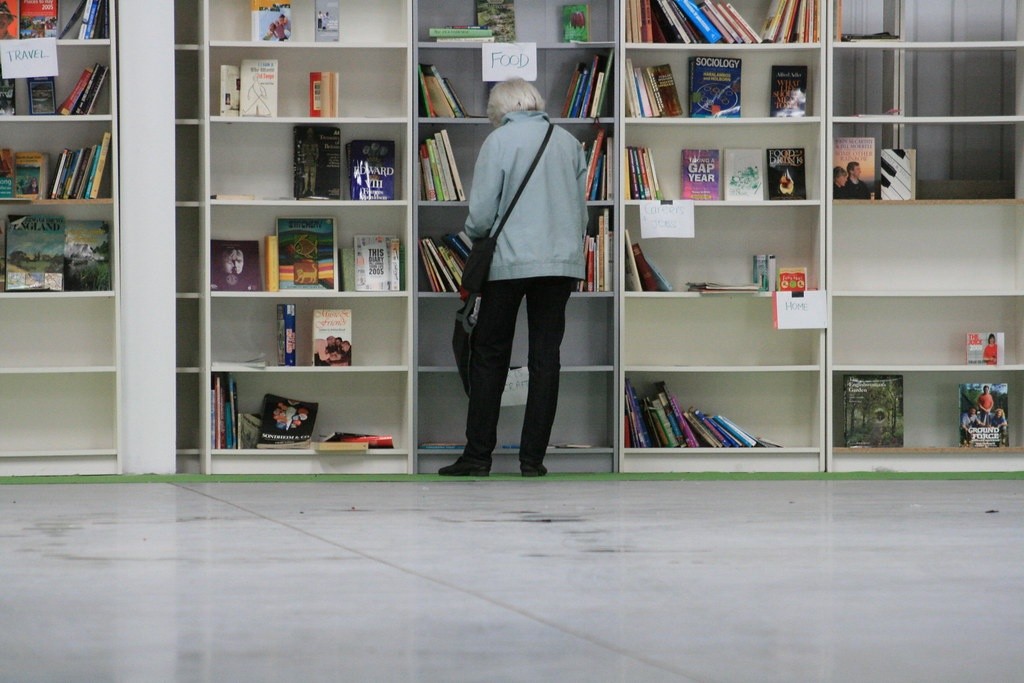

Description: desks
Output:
[0,0,205,483]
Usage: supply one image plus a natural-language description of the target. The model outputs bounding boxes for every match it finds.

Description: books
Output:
[418,0,614,447]
[312,308,352,366]
[833,137,875,200]
[211,376,394,451]
[251,0,291,41]
[213,304,296,366]
[315,0,339,42]
[0,0,111,292]
[625,0,822,448]
[959,383,1008,447]
[211,58,406,291]
[833,0,901,42]
[843,375,903,448]
[881,148,917,200]
[967,332,1004,365]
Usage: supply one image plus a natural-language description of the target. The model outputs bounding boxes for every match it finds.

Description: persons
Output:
[313,336,352,366]
[833,162,871,200]
[263,14,288,41]
[0,3,13,40]
[983,334,997,365]
[438,78,587,478]
[960,385,1007,447]
[212,249,256,290]
[318,11,329,29]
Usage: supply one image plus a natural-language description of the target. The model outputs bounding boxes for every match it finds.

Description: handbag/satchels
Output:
[451,292,478,397]
[461,237,494,292]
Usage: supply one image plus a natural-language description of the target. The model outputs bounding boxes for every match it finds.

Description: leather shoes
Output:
[439,456,490,476]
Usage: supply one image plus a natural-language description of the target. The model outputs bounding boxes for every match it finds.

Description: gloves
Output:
[460,287,470,301]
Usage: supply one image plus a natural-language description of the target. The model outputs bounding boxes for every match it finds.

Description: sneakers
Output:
[520,462,547,476]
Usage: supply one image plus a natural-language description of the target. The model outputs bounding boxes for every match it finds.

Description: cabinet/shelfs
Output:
[204,0,413,481]
[827,0,1024,481]
[414,0,619,482]
[619,0,824,480]
